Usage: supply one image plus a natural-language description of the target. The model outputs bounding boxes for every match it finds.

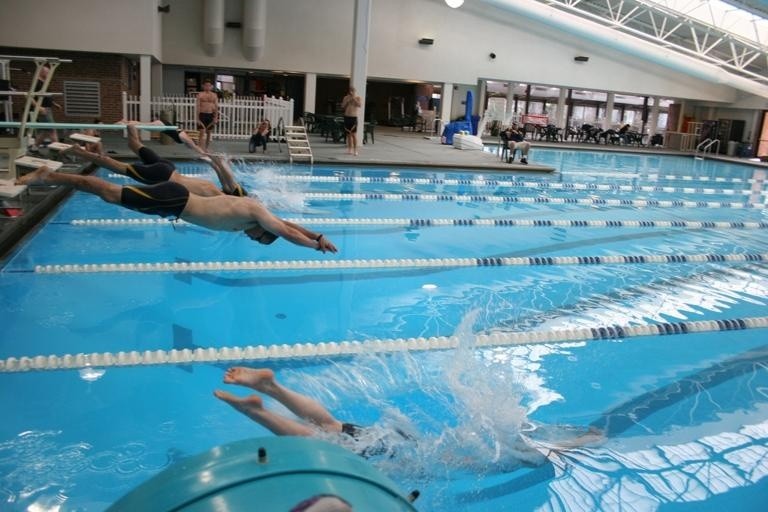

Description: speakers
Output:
[225,22,240,27]
[575,57,588,61]
[422,38,433,44]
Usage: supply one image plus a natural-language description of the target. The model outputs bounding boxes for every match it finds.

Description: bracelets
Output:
[316,233,323,241]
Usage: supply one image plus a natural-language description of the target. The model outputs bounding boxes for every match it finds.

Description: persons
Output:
[200,152,248,197]
[111,118,204,153]
[13,166,338,256]
[617,124,630,145]
[499,120,530,164]
[341,86,361,155]
[30,67,61,145]
[78,119,108,156]
[248,119,270,152]
[212,367,604,472]
[57,142,226,196]
[410,101,423,115]
[127,123,159,163]
[196,78,218,146]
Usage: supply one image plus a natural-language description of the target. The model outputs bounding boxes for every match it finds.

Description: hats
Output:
[512,120,519,125]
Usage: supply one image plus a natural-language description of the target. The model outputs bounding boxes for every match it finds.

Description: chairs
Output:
[303,108,378,146]
[500,130,525,162]
[533,121,650,147]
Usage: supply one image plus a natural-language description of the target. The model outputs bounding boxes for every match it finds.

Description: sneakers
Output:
[508,156,529,164]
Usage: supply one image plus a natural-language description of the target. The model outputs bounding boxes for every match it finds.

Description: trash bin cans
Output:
[160,110,177,145]
[727,141,739,156]
